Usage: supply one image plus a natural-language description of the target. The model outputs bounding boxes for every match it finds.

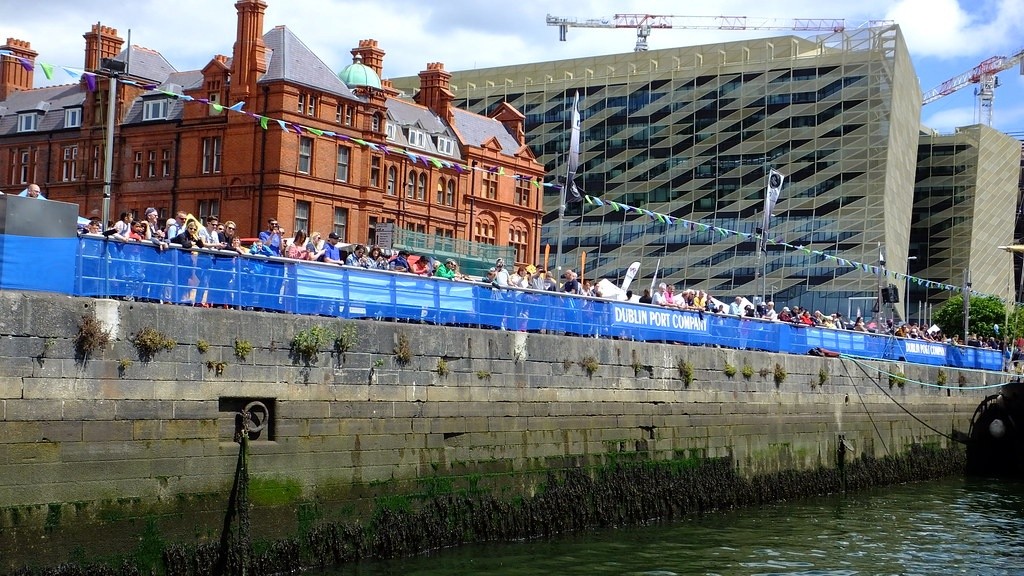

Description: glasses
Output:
[228,226,235,231]
[211,224,218,227]
[269,223,277,226]
[189,227,196,231]
[446,262,453,266]
[219,228,225,231]
[538,267,543,269]
[179,218,186,221]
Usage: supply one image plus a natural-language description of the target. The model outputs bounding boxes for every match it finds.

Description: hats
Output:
[145,207,154,216]
[329,232,341,239]
[446,258,454,265]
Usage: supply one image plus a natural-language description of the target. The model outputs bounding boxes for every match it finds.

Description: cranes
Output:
[921,47,1024,129]
[545,11,895,53]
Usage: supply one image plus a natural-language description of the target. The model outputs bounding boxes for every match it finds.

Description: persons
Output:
[19,179,464,327]
[476,257,1024,373]
